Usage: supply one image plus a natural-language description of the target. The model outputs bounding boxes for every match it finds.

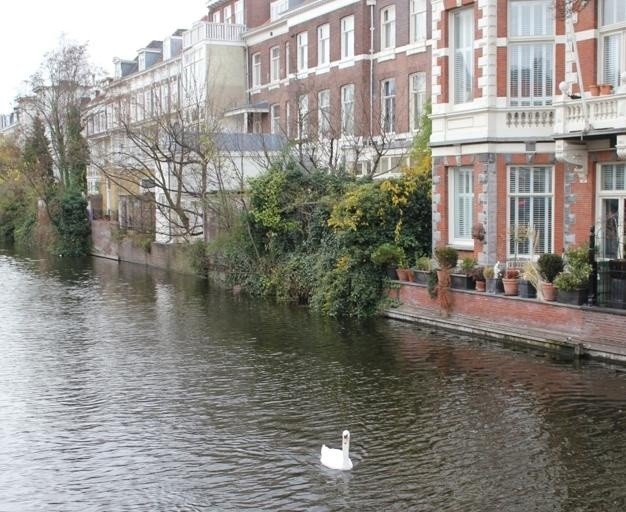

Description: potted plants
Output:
[370,238,600,306]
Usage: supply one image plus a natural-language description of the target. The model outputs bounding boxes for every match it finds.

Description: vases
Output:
[600,84,612,95]
[589,85,600,96]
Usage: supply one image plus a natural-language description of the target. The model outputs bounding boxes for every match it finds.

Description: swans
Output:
[319,429,353,472]
